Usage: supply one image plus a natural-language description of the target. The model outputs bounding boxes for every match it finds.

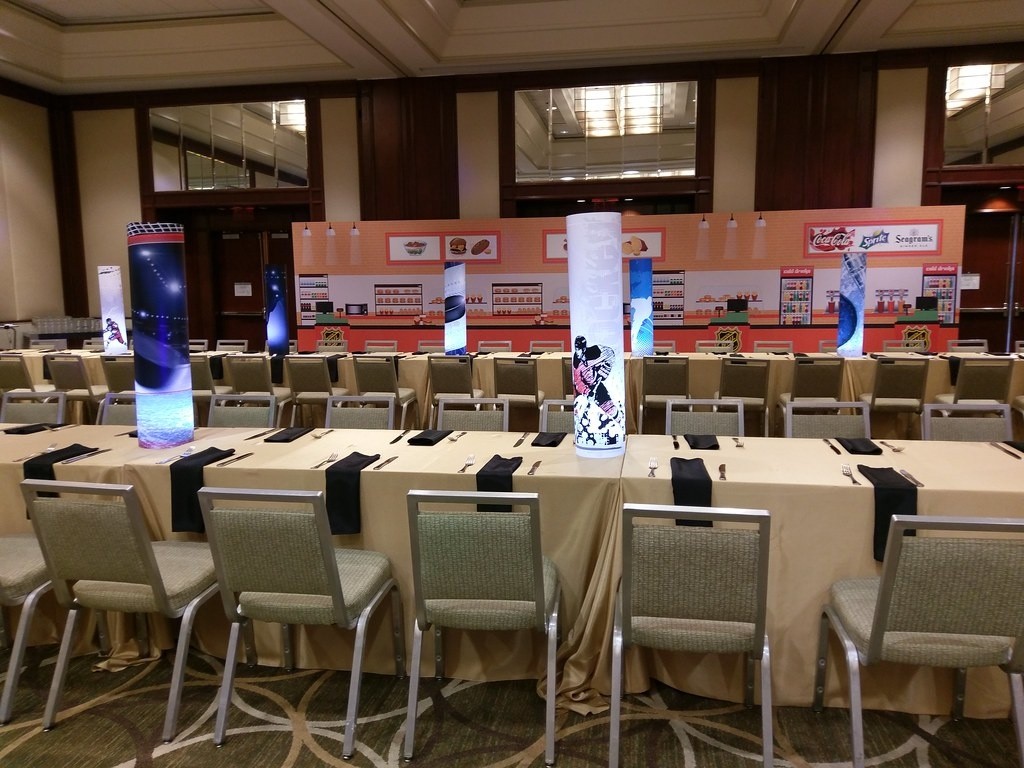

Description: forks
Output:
[310,452,338,469]
[732,437,745,447]
[13,442,61,462]
[447,431,467,441]
[880,441,906,452]
[311,429,334,439]
[842,464,861,485]
[457,455,475,474]
[42,424,81,431]
[647,456,658,477]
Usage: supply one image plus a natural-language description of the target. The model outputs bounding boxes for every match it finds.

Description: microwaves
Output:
[623,303,631,315]
[345,303,368,316]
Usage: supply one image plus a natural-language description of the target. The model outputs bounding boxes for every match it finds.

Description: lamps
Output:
[946,65,1006,108]
[279,100,306,133]
[571,83,664,137]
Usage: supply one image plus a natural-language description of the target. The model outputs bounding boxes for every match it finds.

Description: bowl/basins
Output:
[403,240,428,256]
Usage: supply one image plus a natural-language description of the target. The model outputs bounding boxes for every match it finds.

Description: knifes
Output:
[62,447,113,464]
[527,460,542,475]
[719,463,726,480]
[373,456,398,469]
[389,428,411,445]
[900,469,924,488]
[216,451,254,467]
[155,446,196,464]
[991,440,1021,460]
[822,438,841,456]
[244,427,281,440]
[513,431,530,447]
[672,434,680,450]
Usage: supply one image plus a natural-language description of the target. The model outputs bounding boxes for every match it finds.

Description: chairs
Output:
[197,487,406,761]
[608,502,774,768]
[19,478,220,746]
[0,532,53,727]
[403,489,561,768]
[0,340,1024,443]
[810,515,1024,768]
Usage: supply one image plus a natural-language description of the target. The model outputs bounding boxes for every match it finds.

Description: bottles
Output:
[302,313,316,319]
[300,278,327,287]
[652,286,683,296]
[783,291,811,302]
[783,280,809,290]
[938,299,952,311]
[925,277,952,288]
[653,300,684,310]
[653,312,682,317]
[782,302,810,313]
[301,289,328,298]
[301,301,311,310]
[925,289,955,299]
[782,314,809,325]
[652,275,684,285]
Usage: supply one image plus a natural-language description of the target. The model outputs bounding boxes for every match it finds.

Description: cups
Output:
[898,300,905,313]
[938,313,952,325]
[32,311,96,332]
[1003,303,1007,309]
[888,300,894,312]
[1015,303,1019,309]
[828,300,835,313]
[878,300,884,313]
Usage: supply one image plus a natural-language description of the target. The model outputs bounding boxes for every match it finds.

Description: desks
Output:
[0,421,233,672]
[0,348,111,425]
[618,435,1024,720]
[124,428,620,716]
[630,353,843,438]
[227,351,432,431]
[81,350,240,427]
[23,329,133,349]
[394,346,574,432]
[828,352,1024,442]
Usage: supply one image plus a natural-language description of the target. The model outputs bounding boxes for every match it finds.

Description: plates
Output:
[470,239,491,257]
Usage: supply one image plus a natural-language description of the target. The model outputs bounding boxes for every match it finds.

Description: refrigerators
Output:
[921,262,958,325]
[779,265,814,325]
